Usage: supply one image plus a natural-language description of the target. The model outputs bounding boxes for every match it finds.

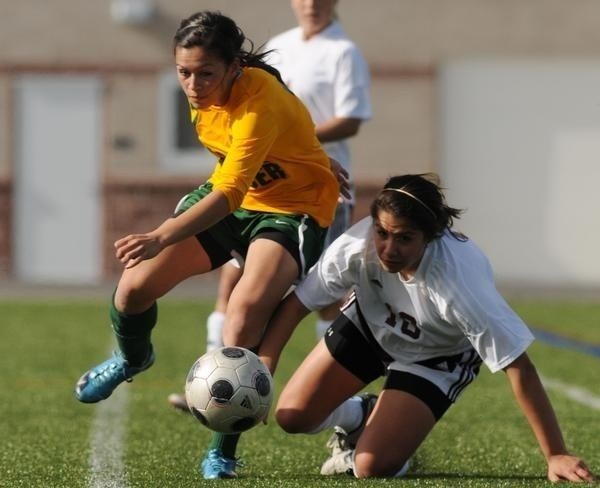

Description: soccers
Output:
[185,346,272,433]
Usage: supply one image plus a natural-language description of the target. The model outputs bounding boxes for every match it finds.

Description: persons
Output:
[154,1,374,415]
[72,8,340,480]
[256,168,595,486]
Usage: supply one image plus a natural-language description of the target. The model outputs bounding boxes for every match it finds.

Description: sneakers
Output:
[168,392,188,410]
[73,347,156,403]
[326,393,378,456]
[201,449,245,479]
[319,449,359,479]
